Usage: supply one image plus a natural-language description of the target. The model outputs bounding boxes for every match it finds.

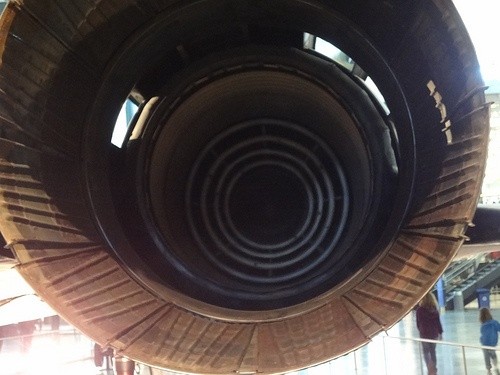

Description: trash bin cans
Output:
[476,288,490,308]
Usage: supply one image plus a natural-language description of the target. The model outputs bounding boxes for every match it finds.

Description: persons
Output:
[101,348,114,371]
[416,292,443,375]
[477,306,500,375]
